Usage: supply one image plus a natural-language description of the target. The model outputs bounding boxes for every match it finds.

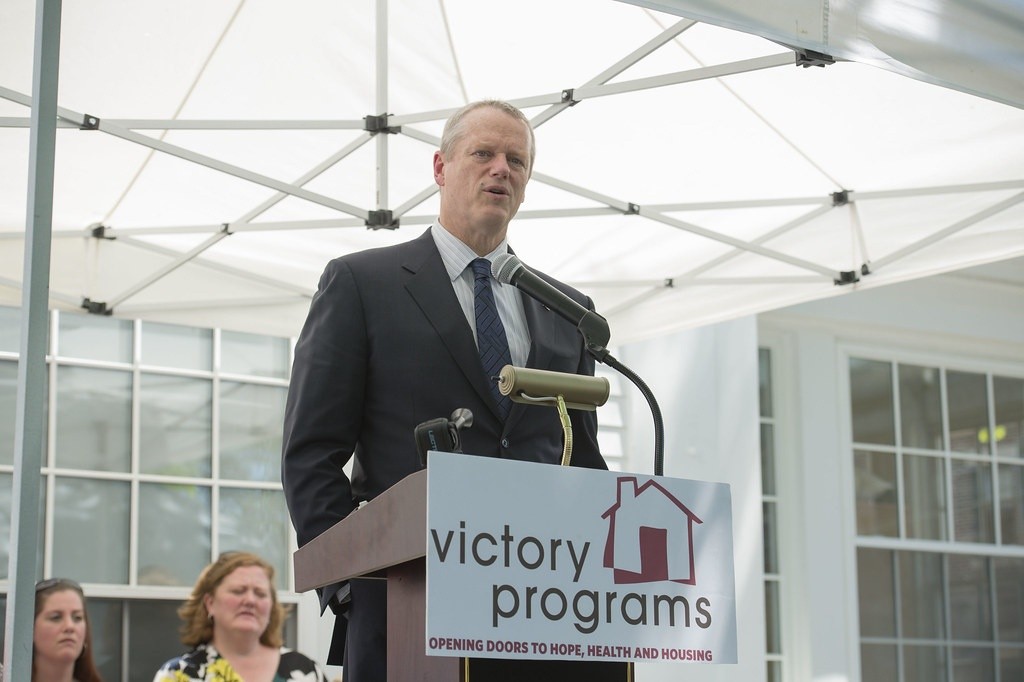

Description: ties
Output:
[468,260,514,423]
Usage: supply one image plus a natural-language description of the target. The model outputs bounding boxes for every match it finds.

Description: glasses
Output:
[34,579,83,595]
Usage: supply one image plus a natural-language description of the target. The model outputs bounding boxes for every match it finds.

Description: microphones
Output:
[492,253,609,337]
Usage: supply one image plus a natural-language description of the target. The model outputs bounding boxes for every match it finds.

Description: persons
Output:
[281,99,609,682]
[32,578,104,682]
[153,551,328,682]
